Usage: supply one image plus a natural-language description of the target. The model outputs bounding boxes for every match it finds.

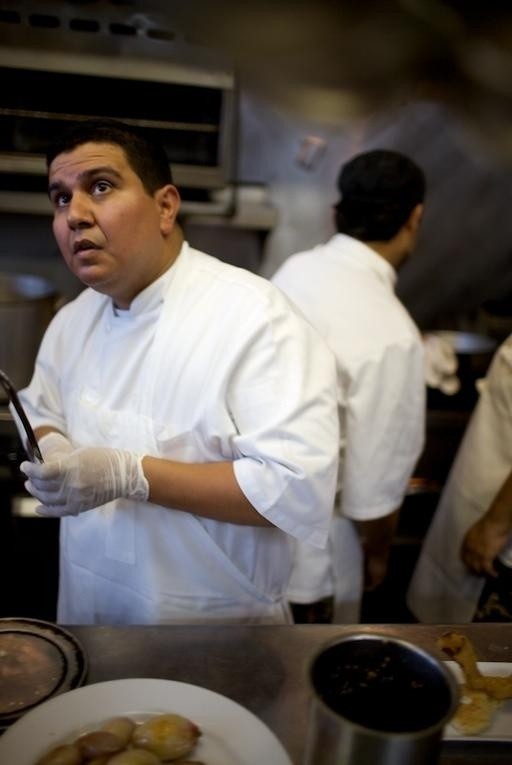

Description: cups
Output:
[302,629,461,765]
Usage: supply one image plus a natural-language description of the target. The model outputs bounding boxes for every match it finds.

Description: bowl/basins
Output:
[210,180,269,207]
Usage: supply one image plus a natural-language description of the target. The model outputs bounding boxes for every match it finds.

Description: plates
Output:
[0,617,90,730]
[0,677,296,764]
[433,659,512,743]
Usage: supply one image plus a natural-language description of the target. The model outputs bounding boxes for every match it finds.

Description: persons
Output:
[269,150,427,623]
[407,338,512,627]
[10,120,340,625]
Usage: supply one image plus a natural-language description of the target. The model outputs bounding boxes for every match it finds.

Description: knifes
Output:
[0,370,45,465]
